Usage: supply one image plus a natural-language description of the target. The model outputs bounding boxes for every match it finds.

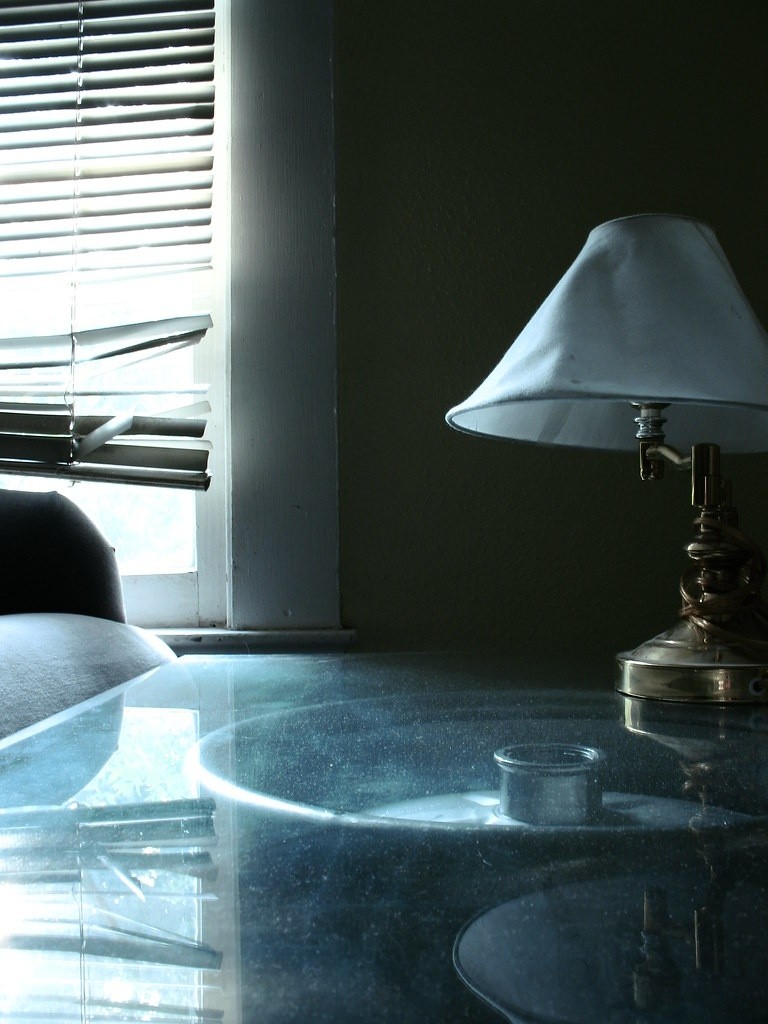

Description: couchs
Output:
[1,488,178,741]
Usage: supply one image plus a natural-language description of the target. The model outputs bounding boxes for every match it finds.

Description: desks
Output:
[1,653,768,1024]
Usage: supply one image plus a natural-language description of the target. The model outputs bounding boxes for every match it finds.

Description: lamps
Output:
[444,213,767,703]
